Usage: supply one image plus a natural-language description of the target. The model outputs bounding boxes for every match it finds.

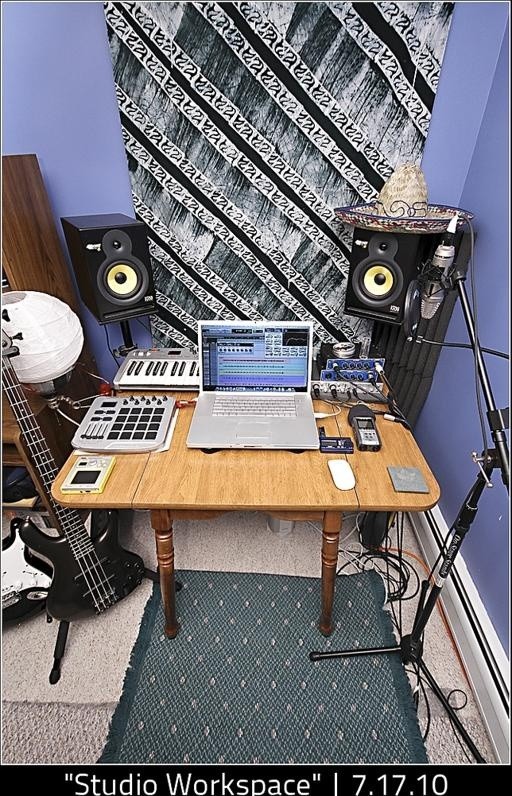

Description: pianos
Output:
[113,350,200,396]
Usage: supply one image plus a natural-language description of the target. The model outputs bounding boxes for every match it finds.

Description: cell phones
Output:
[353,416,380,446]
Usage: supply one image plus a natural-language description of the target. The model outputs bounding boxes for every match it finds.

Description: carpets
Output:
[89,568,434,764]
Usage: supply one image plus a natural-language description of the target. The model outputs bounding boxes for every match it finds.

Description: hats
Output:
[334,165,473,234]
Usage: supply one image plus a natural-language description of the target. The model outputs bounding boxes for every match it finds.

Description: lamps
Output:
[1,290,111,427]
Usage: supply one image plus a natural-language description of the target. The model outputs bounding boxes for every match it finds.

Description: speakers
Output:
[60,213,156,324]
[345,221,458,322]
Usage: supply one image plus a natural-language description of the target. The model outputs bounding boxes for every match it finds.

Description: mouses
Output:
[328,460,356,491]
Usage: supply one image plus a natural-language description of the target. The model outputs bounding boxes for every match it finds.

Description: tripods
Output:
[308,450,492,765]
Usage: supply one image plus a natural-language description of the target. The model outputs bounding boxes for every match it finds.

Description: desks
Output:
[47,394,442,643]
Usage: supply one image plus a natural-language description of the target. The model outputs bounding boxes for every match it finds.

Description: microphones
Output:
[419,215,459,319]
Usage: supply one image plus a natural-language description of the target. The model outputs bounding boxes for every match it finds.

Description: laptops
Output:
[186,320,320,452]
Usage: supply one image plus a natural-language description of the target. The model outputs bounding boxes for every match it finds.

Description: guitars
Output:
[1,327,144,623]
